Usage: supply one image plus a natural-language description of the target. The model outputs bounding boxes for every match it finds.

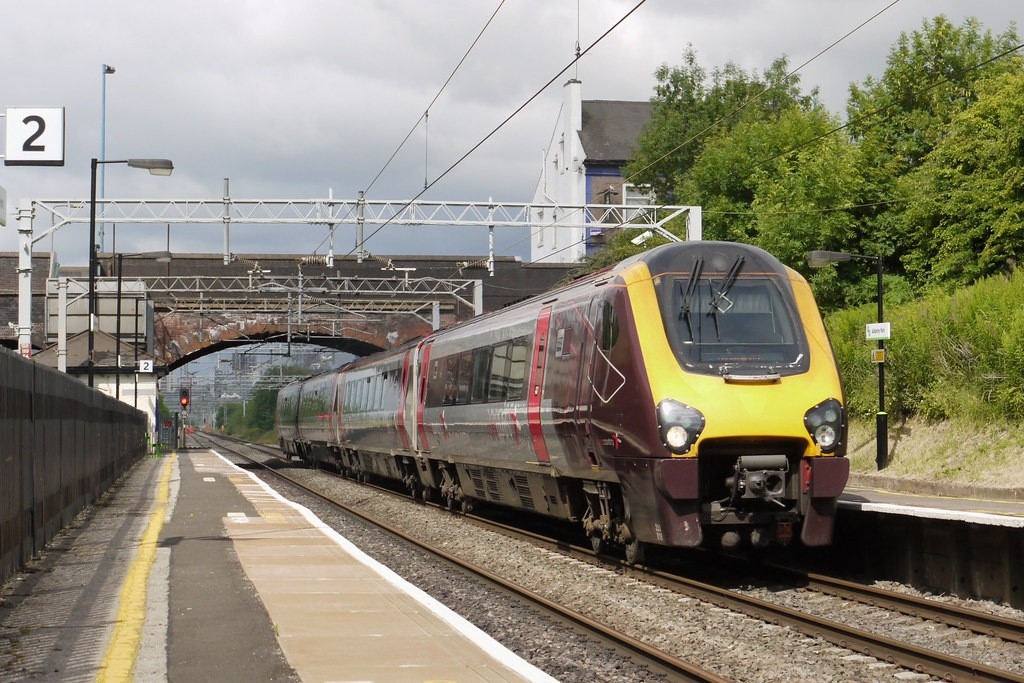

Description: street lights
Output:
[134,296,175,409]
[86,157,173,388]
[803,249,889,471]
[116,251,171,400]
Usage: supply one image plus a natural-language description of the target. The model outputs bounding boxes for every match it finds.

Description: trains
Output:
[274,240,850,568]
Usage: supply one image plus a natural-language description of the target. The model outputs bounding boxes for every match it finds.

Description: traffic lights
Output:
[179,389,190,408]
